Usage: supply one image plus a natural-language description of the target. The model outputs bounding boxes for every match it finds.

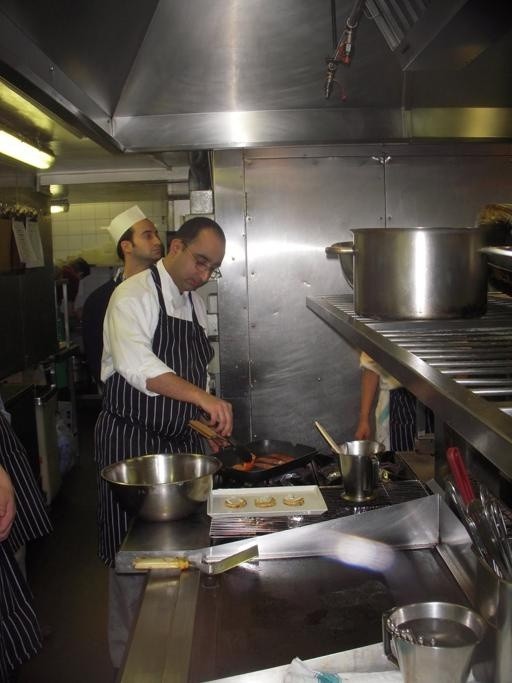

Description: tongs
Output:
[199,407,255,464]
[443,474,512,586]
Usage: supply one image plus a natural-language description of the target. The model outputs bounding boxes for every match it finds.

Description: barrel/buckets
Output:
[335,438,385,505]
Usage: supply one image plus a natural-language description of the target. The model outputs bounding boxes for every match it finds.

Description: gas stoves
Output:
[206,438,416,492]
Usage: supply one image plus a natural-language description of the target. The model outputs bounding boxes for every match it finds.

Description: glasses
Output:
[183,244,223,281]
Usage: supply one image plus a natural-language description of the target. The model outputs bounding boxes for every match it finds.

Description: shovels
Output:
[446,446,510,582]
[132,544,259,576]
[188,417,252,464]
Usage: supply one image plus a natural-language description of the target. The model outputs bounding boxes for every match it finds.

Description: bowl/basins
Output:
[99,451,223,525]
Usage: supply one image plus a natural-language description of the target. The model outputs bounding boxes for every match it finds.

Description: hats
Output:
[107,202,147,247]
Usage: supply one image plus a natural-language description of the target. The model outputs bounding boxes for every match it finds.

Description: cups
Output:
[330,437,386,502]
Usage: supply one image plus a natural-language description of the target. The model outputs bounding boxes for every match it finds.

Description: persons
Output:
[0,462,43,682]
[355,350,434,463]
[81,204,163,382]
[1,397,53,581]
[90,217,235,682]
[54,257,90,318]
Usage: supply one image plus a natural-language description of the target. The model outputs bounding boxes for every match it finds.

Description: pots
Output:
[182,417,321,483]
[324,222,490,319]
